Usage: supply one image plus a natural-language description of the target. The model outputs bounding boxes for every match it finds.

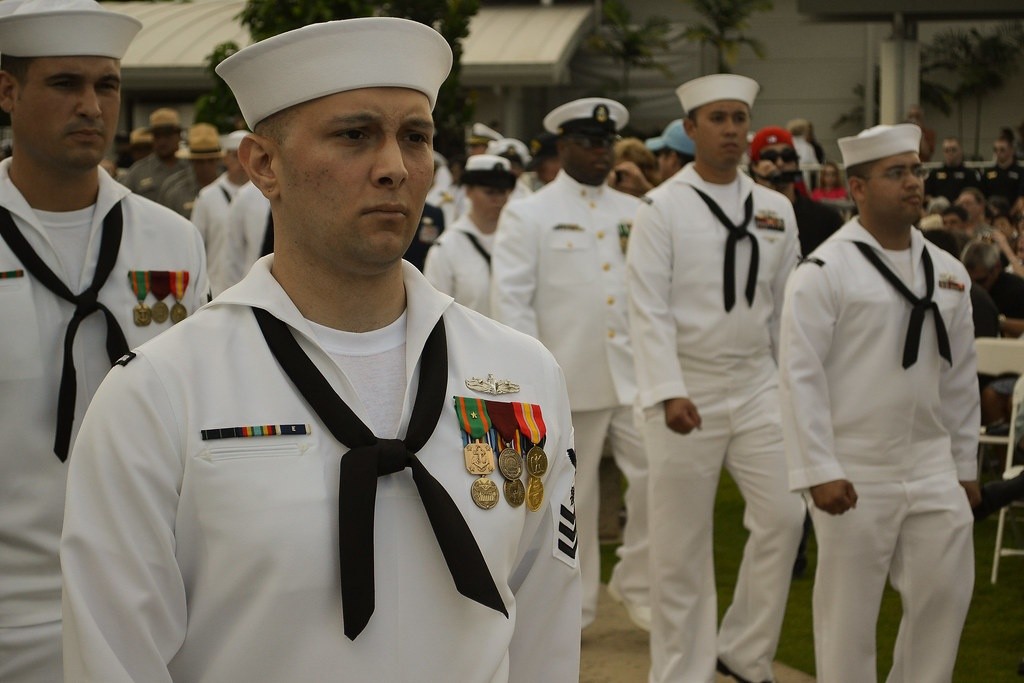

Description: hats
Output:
[486,137,532,168]
[226,129,254,152]
[676,73,761,113]
[175,123,226,160]
[465,123,504,146]
[542,97,630,138]
[126,129,155,148]
[461,155,516,188]
[0,1,143,60]
[751,127,795,160]
[837,123,922,169]
[215,17,454,135]
[142,109,185,132]
[646,118,695,156]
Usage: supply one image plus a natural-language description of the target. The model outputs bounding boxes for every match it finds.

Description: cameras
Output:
[768,170,803,190]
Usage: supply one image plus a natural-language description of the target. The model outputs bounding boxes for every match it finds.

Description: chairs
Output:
[971,336,1023,585]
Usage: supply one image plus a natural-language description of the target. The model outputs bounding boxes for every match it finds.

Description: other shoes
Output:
[606,576,651,634]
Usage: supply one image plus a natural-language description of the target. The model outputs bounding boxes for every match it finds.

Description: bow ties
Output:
[854,243,953,368]
[688,187,762,313]
[0,202,132,466]
[250,306,508,639]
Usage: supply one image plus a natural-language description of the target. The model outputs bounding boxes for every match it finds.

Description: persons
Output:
[0,0,215,683]
[626,73,806,683]
[488,97,663,633]
[99,106,1024,548]
[59,14,584,683]
[776,123,982,683]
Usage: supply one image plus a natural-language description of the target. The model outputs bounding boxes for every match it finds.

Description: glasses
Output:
[760,148,798,165]
[568,136,618,149]
